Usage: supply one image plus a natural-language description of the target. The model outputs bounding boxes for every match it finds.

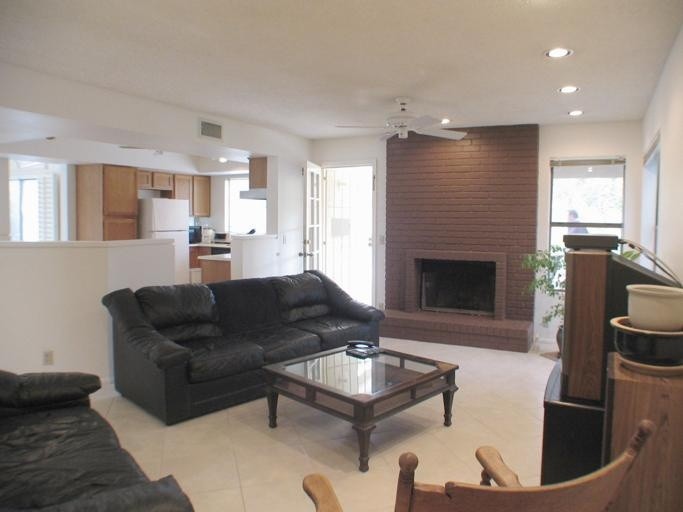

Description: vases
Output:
[608,316,683,377]
[626,281,683,332]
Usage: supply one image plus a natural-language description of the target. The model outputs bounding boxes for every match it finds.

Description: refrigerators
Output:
[139,198,189,275]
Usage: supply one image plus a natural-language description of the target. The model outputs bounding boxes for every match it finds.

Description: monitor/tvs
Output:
[559,249,683,406]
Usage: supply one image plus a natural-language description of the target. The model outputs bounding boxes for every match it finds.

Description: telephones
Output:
[345,340,378,358]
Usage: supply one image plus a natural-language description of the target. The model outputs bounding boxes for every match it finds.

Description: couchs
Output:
[1,368,194,512]
[102,271,385,426]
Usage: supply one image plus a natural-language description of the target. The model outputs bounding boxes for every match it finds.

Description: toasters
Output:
[202,228,216,244]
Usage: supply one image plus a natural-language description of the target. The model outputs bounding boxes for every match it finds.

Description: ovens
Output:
[189,226,201,244]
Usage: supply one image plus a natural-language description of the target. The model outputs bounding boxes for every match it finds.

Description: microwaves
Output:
[213,232,230,242]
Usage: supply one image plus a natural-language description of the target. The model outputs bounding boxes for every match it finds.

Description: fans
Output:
[335,97,468,142]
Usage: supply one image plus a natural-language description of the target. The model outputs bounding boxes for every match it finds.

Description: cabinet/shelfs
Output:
[541,342,604,485]
[76,164,136,218]
[136,170,174,192]
[161,175,211,217]
[248,156,267,189]
[231,234,302,280]
[199,248,211,267]
[190,246,199,269]
[77,215,137,240]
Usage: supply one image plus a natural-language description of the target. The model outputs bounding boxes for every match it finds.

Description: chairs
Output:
[302,419,654,512]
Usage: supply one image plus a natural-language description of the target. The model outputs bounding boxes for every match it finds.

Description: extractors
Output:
[239,188,268,201]
